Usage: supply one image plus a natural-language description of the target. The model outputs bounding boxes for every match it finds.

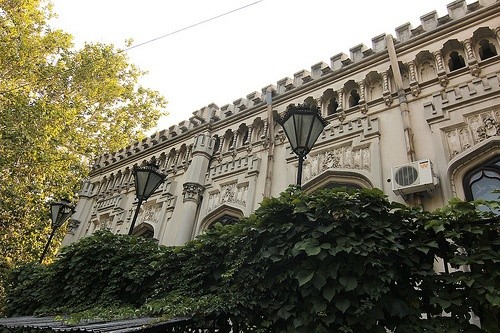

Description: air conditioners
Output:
[390,158,440,196]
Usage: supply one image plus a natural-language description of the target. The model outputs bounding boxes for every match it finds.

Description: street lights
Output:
[276,102,331,192]
[36,194,76,264]
[128,154,169,236]
